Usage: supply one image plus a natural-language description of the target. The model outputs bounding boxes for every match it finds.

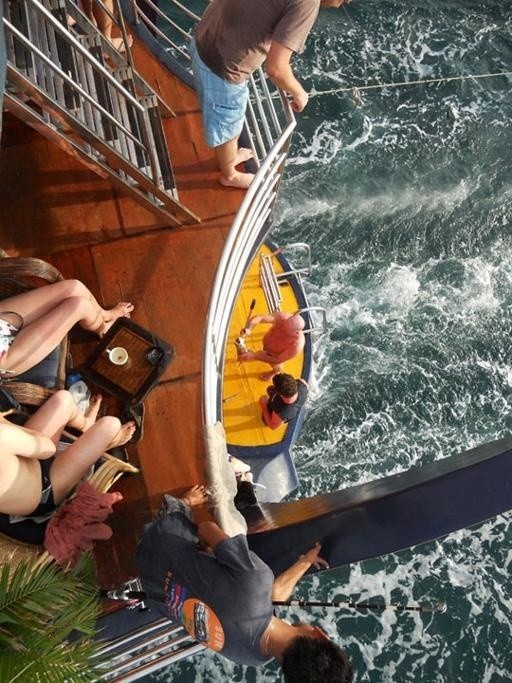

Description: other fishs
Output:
[233,335,249,367]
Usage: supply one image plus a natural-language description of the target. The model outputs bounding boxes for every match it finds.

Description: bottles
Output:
[228,452,251,473]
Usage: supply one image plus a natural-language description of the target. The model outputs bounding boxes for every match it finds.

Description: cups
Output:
[106,346,129,366]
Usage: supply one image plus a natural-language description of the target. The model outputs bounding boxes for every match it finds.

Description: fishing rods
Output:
[272,601,448,615]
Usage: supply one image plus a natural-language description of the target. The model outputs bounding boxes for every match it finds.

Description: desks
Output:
[84,314,176,406]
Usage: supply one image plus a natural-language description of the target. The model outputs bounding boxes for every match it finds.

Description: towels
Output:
[41,482,125,572]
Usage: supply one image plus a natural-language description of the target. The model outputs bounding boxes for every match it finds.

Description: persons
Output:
[134,482,351,682]
[258,371,309,430]
[1,392,137,518]
[1,278,133,378]
[192,0,344,190]
[82,0,132,53]
[234,312,305,380]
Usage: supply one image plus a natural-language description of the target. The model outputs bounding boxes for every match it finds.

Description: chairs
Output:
[0,408,141,586]
[0,253,71,406]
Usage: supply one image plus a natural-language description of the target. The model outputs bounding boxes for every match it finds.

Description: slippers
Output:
[121,402,146,443]
